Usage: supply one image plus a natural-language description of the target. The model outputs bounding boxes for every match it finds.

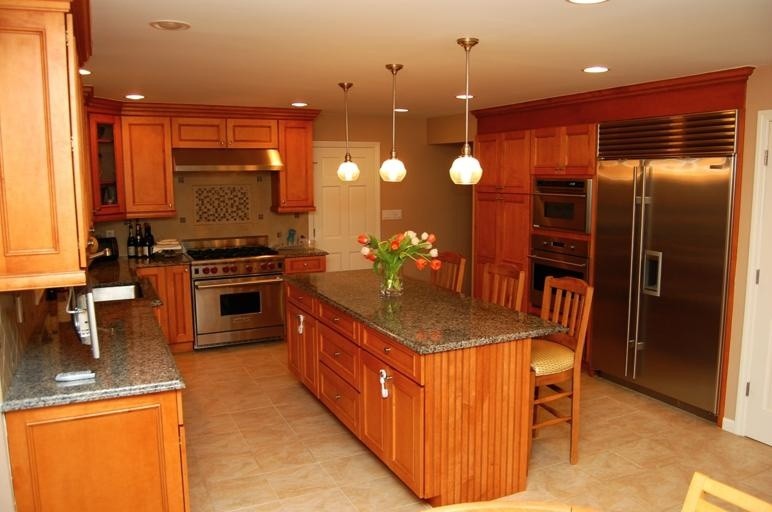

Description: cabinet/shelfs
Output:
[469,125,594,376]
[359,326,425,500]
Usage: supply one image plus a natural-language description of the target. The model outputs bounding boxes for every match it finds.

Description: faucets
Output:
[88,248,113,260]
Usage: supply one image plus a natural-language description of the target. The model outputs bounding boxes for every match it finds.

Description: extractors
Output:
[168,145,286,171]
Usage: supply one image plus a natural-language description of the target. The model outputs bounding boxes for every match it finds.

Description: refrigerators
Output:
[591,158,737,424]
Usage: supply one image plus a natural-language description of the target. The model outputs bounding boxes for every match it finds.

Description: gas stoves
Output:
[187,246,280,279]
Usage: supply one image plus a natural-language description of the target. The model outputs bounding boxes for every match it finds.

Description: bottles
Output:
[287,228,296,247]
[143,223,154,258]
[127,225,135,258]
[134,223,143,258]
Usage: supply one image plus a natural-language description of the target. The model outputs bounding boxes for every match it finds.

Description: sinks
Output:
[91,283,145,303]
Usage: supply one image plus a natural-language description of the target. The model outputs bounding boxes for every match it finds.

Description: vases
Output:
[377,262,405,298]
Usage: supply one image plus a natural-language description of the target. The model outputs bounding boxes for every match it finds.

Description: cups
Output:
[306,239,316,250]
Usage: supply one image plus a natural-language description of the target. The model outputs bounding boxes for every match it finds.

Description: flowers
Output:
[356,230,443,290]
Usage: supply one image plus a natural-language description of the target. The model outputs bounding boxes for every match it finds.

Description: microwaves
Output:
[532,174,586,232]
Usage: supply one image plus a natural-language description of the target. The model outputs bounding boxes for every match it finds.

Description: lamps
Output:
[336,82,361,183]
[448,36,484,187]
[379,63,407,183]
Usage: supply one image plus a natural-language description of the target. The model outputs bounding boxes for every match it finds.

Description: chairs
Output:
[429,251,595,466]
[681,472,772,511]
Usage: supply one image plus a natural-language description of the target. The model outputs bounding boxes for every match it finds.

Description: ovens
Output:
[193,275,285,351]
[529,234,588,316]
